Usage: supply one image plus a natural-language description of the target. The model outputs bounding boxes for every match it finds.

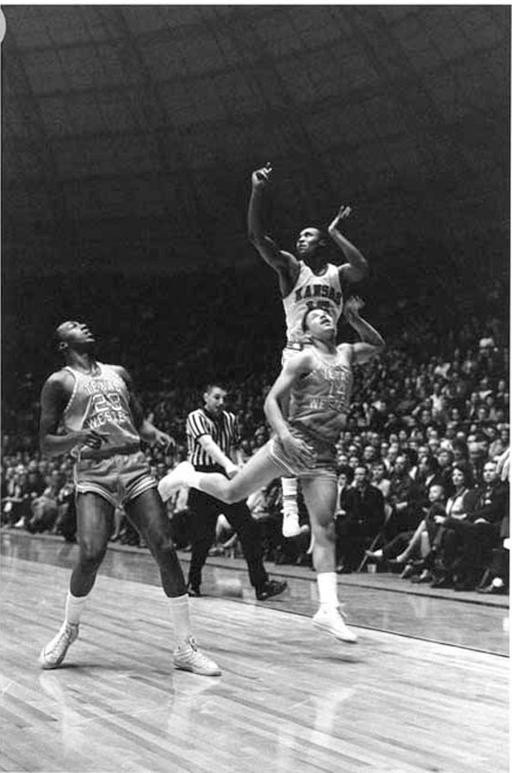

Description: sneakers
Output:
[185,579,200,598]
[38,620,81,671]
[310,606,359,644]
[334,549,509,595]
[170,633,222,677]
[256,579,288,601]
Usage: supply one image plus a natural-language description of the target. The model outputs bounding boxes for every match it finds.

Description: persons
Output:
[155,292,389,645]
[2,300,510,600]
[244,160,371,538]
[183,380,289,601]
[37,316,225,678]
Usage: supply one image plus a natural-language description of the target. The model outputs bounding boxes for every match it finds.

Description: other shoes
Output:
[0,517,77,542]
[111,526,145,550]
[209,538,244,559]
[171,538,193,553]
[262,542,312,566]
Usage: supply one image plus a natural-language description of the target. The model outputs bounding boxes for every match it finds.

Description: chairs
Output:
[350,503,393,572]
